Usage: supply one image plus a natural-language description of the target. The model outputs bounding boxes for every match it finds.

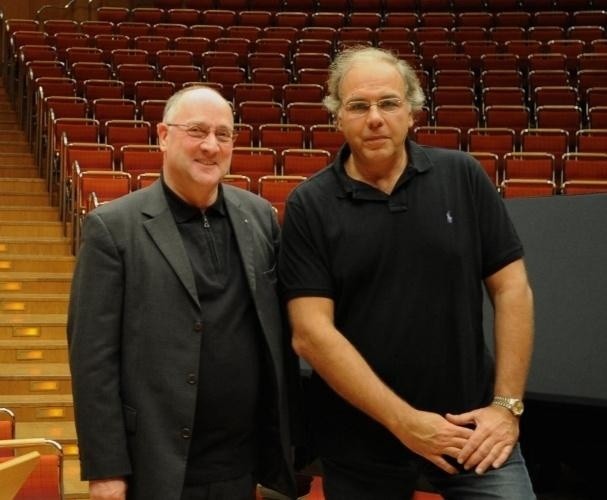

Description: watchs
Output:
[491,396,524,416]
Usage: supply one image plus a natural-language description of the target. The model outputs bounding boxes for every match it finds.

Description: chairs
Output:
[0,1,606,257]
[0,435,67,500]
[0,402,19,457]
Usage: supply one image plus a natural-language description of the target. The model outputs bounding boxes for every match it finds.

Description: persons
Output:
[277,46,538,500]
[66,85,314,500]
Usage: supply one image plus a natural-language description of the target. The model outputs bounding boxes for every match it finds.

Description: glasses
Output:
[340,98,409,119]
[167,123,238,142]
